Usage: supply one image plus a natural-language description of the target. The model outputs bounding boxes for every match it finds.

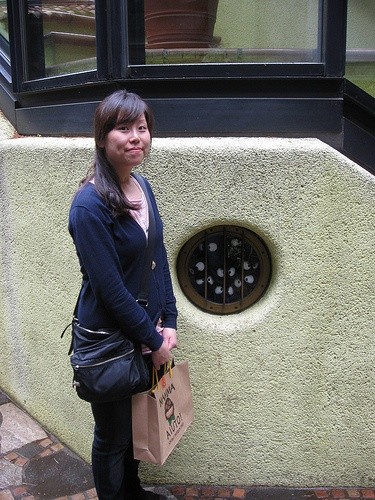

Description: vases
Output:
[143,0,220,49]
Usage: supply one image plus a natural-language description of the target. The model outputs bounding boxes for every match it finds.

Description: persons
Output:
[67,90,178,500]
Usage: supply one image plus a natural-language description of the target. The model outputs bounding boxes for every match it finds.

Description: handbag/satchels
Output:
[131,357,197,465]
[67,316,151,403]
